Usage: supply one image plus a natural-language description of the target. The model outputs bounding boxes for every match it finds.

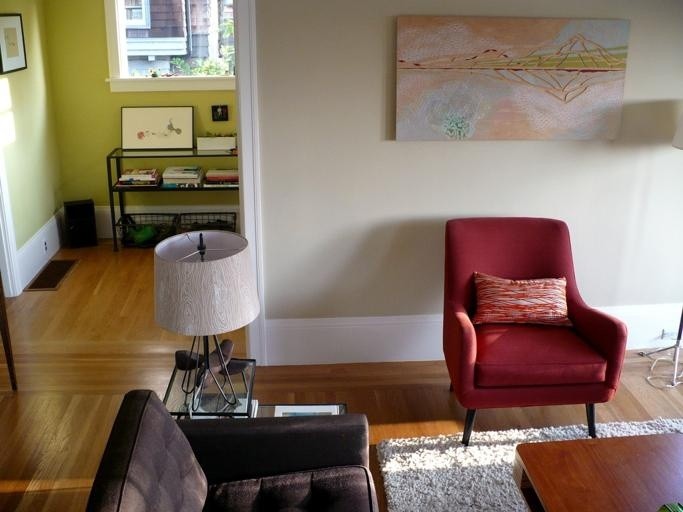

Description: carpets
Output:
[24,259,79,292]
[379,418,682,511]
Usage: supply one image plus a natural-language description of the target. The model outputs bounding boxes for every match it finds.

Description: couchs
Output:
[83,391,377,511]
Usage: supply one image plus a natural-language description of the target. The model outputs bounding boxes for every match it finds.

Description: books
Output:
[189,397,259,420]
[115,165,239,189]
[273,405,340,418]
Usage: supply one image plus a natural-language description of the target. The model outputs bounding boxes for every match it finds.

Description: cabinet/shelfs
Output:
[107,147,238,252]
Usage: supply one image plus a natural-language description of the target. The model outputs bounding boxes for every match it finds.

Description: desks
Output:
[161,354,256,420]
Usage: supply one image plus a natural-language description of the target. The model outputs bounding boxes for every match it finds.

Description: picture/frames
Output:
[0,13,27,75]
[120,105,194,150]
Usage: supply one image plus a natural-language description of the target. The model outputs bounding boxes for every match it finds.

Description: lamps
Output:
[154,229,260,408]
[646,115,683,390]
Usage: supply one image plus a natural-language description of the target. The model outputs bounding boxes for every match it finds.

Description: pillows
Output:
[473,270,570,325]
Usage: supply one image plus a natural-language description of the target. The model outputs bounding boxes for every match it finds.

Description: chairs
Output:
[444,216,628,444]
